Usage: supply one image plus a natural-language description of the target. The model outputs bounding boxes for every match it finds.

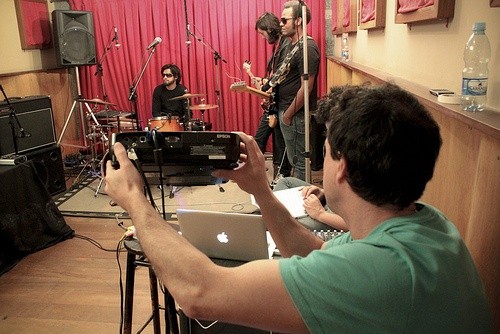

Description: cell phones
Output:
[430,88,453,96]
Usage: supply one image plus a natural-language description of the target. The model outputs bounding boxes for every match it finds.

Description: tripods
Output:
[57,65,110,197]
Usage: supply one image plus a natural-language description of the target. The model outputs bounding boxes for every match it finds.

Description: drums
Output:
[148,115,185,132]
[108,122,140,149]
[101,151,113,178]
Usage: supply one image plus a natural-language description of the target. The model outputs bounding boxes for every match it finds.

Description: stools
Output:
[123,223,183,334]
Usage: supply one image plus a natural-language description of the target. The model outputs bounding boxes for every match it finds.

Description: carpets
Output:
[55,152,274,217]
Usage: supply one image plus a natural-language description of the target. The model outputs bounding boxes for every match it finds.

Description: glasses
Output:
[162,73,174,78]
[281,16,301,25]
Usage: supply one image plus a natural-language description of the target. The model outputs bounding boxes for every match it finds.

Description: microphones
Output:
[114,27,120,47]
[147,37,161,50]
[186,23,191,45]
[17,134,31,137]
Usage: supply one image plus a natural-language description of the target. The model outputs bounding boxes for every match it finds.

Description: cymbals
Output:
[185,104,219,110]
[169,93,206,100]
[75,98,119,106]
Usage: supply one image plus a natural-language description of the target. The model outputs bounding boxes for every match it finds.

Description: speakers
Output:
[23,145,67,196]
[51,10,97,66]
[0,94,57,159]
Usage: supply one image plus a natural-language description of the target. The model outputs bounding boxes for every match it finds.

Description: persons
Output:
[272,84,350,232]
[254,12,293,180]
[151,64,193,123]
[274,0,320,182]
[105,83,494,334]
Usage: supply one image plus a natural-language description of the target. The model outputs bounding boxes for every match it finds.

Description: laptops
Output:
[175,209,276,262]
[250,186,310,219]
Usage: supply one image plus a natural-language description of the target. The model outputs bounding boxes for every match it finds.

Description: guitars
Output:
[243,60,278,127]
[230,80,271,99]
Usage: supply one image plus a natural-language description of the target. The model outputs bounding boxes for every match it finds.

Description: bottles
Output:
[460,21,491,112]
[341,37,349,62]
